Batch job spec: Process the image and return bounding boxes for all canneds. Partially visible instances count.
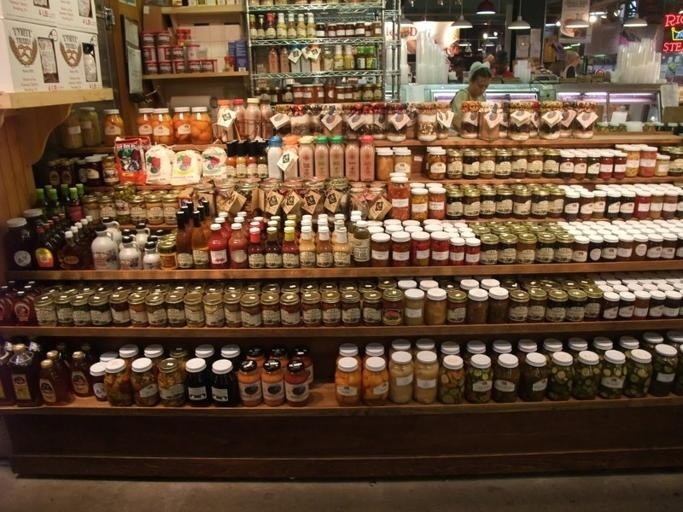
[255,81,597,142]
[5,143,683,407]
[141,29,247,74]
[55,106,213,147]
[315,22,382,39]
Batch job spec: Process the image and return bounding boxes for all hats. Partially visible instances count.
[468,60,491,81]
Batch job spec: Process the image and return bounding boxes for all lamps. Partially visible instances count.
[449,0,647,49]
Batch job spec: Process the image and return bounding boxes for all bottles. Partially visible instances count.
[0,336,93,408]
[48,155,79,187]
[33,181,85,220]
[268,44,376,73]
[174,200,370,270]
[247,12,315,39]
[325,79,335,103]
[31,212,162,272]
[0,280,41,327]
[254,84,292,103]
[226,134,376,184]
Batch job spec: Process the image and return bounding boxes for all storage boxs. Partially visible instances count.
[661,105,682,123]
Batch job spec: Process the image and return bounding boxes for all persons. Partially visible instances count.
[559,49,581,78]
[490,50,514,77]
[450,60,494,110]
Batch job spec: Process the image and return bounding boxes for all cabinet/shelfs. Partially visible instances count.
[140,4,248,78]
[249,2,384,88]
[0,132,683,418]
[400,84,660,128]
